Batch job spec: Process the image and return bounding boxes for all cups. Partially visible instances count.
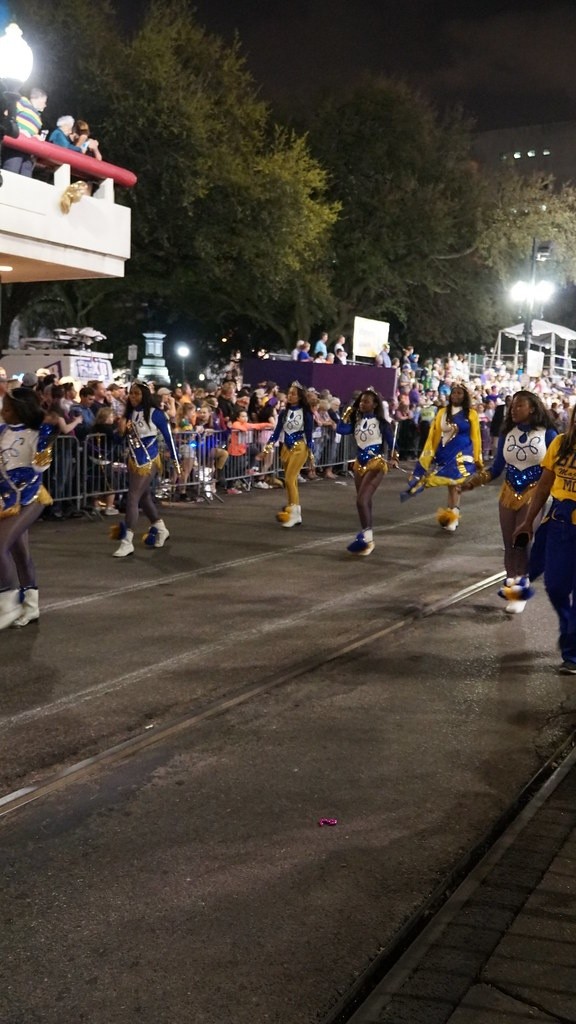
[75,410,82,424]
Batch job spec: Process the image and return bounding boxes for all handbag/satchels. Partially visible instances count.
[112,462,127,473]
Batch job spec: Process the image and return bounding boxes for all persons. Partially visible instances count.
[512,403,576,675]
[456,390,560,614]
[399,384,484,531]
[112,379,181,557]
[335,390,400,555]
[0,388,55,630]
[0,331,576,520]
[3,88,102,195]
[256,380,316,527]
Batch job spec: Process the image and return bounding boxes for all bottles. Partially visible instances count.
[181,417,189,426]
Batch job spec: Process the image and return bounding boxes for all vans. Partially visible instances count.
[1,326,116,389]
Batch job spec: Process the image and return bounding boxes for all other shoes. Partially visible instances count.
[90,506,103,515]
[559,661,576,674]
[155,473,338,503]
[105,507,119,515]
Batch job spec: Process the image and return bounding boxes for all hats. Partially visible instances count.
[30,88,47,99]
[157,388,173,396]
[107,383,123,391]
[22,372,38,386]
[36,368,50,377]
[56,115,75,126]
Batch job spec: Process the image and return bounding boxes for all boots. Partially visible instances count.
[109,522,134,558]
[143,518,169,548]
[347,529,377,556]
[0,587,25,630]
[438,507,460,532]
[497,576,534,614]
[10,585,39,629]
[275,504,302,529]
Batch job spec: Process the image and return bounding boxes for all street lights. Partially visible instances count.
[511,236,557,374]
[175,345,190,383]
